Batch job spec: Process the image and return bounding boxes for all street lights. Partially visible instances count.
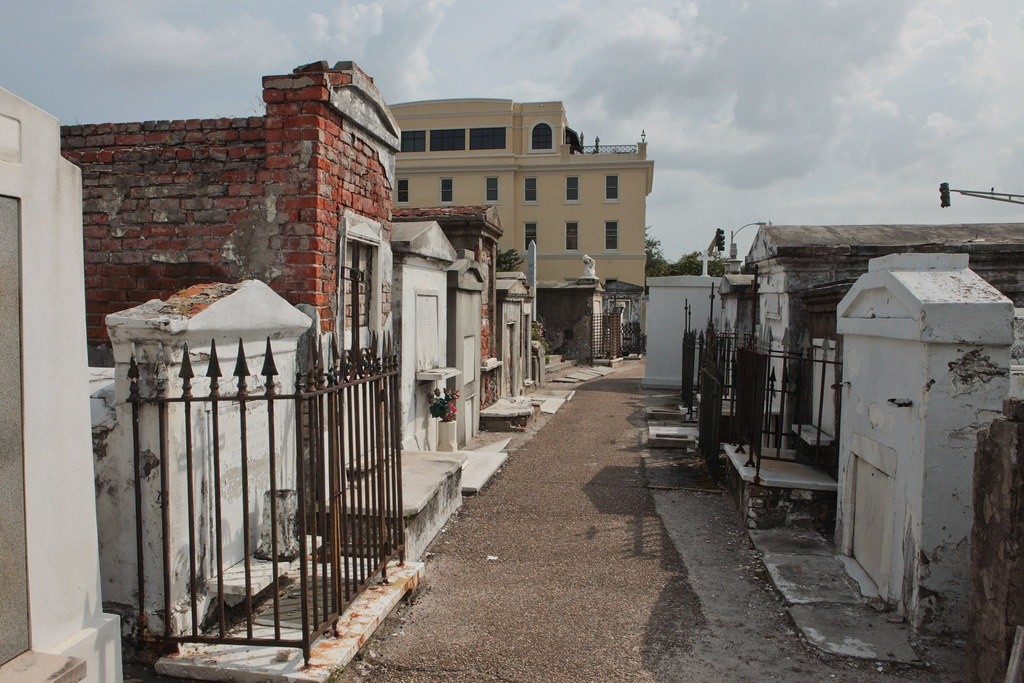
[731,220,772,258]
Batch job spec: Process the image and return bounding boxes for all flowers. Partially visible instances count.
[427,387,461,421]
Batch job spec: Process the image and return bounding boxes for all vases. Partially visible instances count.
[437,420,457,452]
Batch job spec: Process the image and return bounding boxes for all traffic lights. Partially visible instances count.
[715,228,725,252]
[939,182,951,208]
